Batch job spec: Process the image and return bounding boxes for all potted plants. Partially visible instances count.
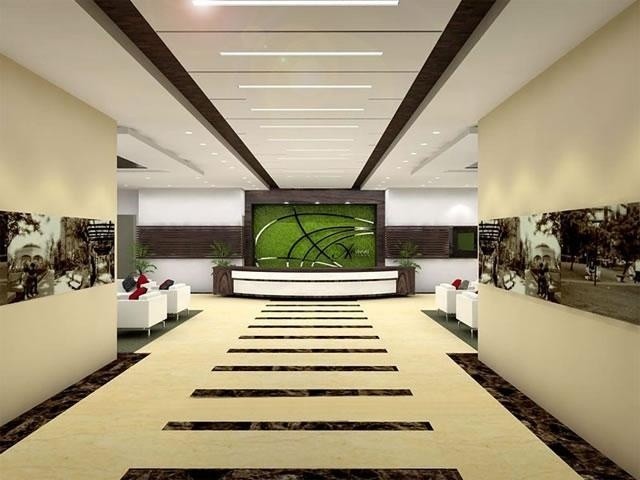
[210,238,235,297]
[391,236,423,296]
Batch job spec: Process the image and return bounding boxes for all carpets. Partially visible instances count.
[420,309,479,353]
[117,309,204,353]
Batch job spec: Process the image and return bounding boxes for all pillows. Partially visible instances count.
[452,278,471,289]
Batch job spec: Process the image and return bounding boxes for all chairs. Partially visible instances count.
[117,277,191,337]
[435,282,478,338]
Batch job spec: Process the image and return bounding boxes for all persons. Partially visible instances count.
[489,241,499,287]
[22,263,39,299]
[589,261,596,280]
[633,255,640,284]
[536,263,550,300]
